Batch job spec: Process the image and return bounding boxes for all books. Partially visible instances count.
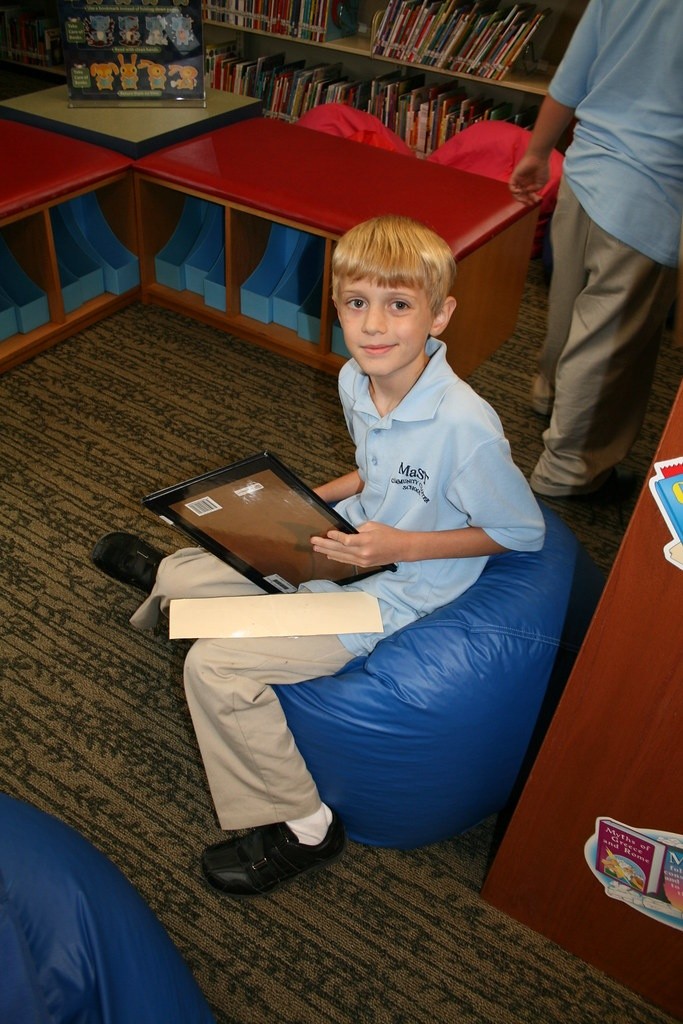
[200,0,553,160]
[0,7,64,67]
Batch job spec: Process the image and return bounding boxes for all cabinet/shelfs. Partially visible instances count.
[1,82,542,388]
[0,1,552,98]
[480,370,683,1024]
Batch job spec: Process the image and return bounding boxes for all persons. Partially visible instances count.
[89,218,545,895]
[508,0,683,496]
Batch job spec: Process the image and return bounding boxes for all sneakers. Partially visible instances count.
[200,801,346,897]
[91,530,173,594]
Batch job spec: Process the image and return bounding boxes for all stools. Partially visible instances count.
[0,791,221,1024]
[265,499,605,849]
[422,118,566,259]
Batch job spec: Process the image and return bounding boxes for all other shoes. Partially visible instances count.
[532,465,636,507]
